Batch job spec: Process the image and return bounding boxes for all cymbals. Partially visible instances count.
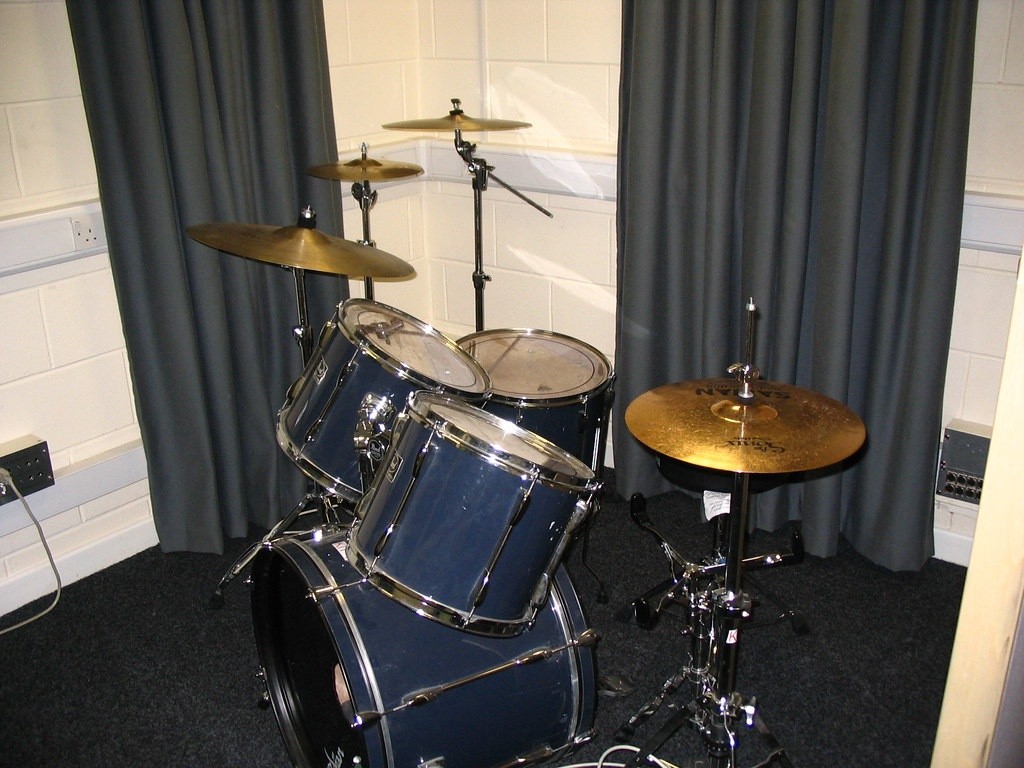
[625,376,867,474]
[381,112,534,133]
[306,158,424,184]
[186,221,414,278]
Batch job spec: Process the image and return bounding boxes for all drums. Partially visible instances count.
[345,389,602,639]
[247,516,600,768]
[449,327,617,480]
[273,299,494,506]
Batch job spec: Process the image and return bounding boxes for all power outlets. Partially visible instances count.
[0,433,55,506]
[70,211,108,251]
